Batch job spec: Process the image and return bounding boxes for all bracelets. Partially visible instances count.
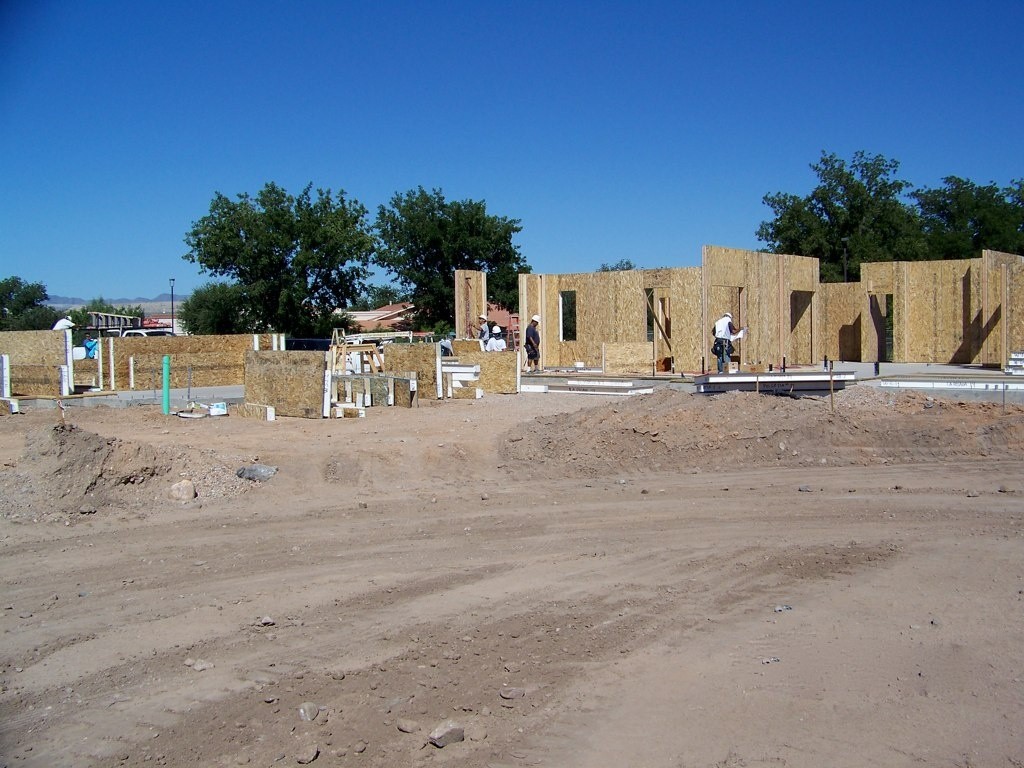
[473,325,475,327]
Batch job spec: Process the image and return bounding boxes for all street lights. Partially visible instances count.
[169,279,175,333]
[841,237,850,282]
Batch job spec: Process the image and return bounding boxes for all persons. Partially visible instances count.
[82,332,98,358]
[449,331,456,340]
[470,314,489,345]
[711,313,744,375]
[51,315,80,330]
[485,325,507,351]
[524,314,541,373]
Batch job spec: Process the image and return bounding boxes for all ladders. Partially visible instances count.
[507,316,519,349]
[87,311,143,330]
[331,328,351,366]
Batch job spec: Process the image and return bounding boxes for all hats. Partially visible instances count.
[724,312,732,318]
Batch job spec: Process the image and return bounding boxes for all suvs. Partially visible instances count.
[122,329,177,337]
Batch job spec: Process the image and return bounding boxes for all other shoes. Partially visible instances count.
[534,370,544,372]
[719,371,723,374]
[525,370,534,374]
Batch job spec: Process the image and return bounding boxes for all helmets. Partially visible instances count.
[479,315,487,321]
[65,316,72,321]
[492,325,501,333]
[532,315,540,323]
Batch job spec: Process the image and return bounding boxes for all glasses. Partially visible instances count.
[85,336,91,337]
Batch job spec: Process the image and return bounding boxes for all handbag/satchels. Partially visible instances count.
[725,345,735,355]
[526,344,531,351]
[712,342,724,357]
